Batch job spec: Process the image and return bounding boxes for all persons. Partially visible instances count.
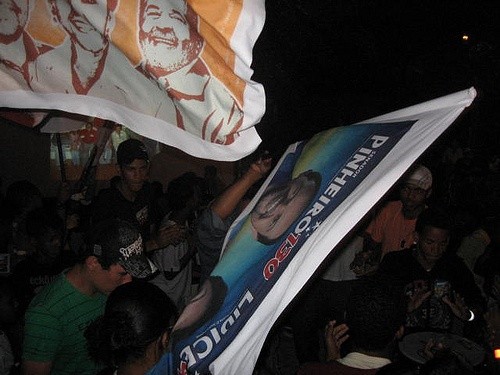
[22,124,271,375]
[292,167,500,375]
[228,143,322,245]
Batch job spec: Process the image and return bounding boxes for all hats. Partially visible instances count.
[407,166,432,191]
[81,217,160,279]
[117,139,149,166]
